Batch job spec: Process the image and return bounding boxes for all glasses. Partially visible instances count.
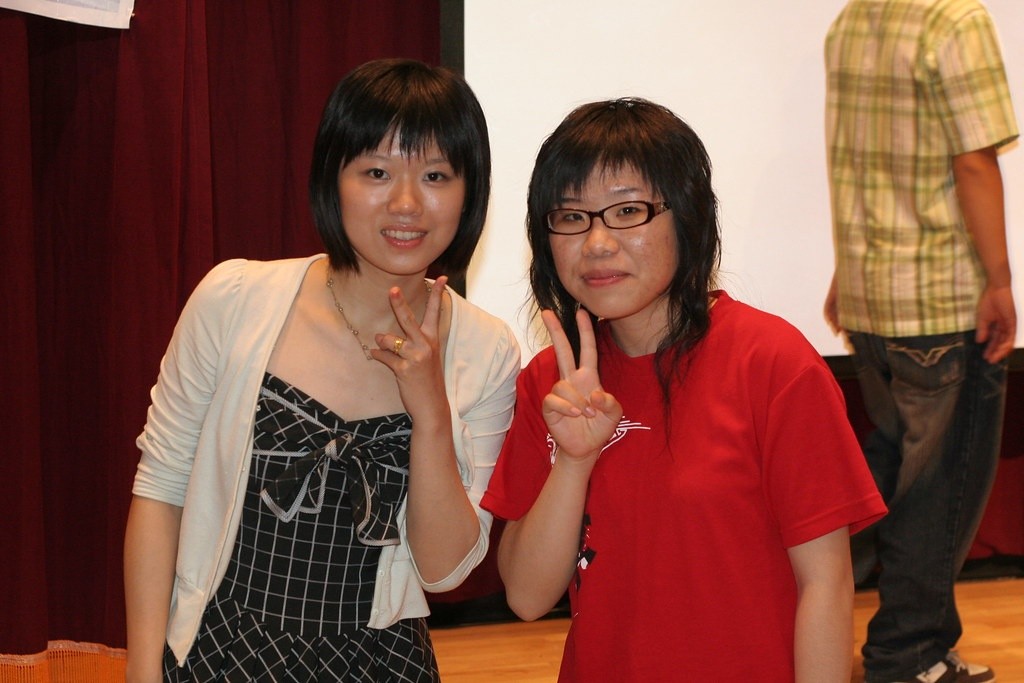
[542,200,673,235]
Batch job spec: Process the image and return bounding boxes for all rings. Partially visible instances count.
[392,337,403,354]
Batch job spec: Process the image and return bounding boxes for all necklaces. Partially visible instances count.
[324,263,431,361]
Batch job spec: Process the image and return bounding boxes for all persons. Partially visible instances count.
[478,97,888,683]
[124,59,522,683]
[823,1,1020,683]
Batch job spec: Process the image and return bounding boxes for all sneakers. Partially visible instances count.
[891,650,997,683]
[854,571,881,591]
